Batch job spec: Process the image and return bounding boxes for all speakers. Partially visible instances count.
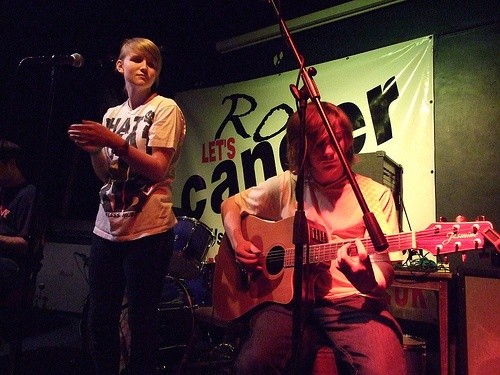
[26,236,97,315]
[448,266,500,375]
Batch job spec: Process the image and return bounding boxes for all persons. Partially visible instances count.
[0,138,45,338]
[66,37,186,375]
[221,102,408,375]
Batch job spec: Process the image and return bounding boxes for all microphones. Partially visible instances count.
[28,53,84,68]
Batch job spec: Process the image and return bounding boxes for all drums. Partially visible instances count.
[167,215,213,281]
[79,268,195,374]
[188,264,216,307]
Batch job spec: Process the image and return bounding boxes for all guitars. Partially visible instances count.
[214,211,500,322]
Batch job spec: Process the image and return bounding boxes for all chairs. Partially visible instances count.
[193,307,248,375]
